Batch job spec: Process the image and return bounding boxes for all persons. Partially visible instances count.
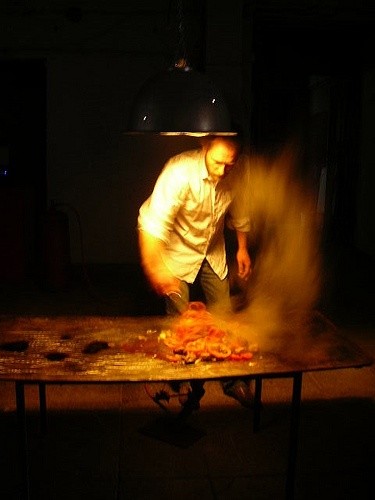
[135,136,253,315]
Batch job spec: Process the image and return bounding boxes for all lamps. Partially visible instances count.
[121,4,237,137]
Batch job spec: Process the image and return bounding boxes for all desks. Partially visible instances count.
[0,309,375,474]
